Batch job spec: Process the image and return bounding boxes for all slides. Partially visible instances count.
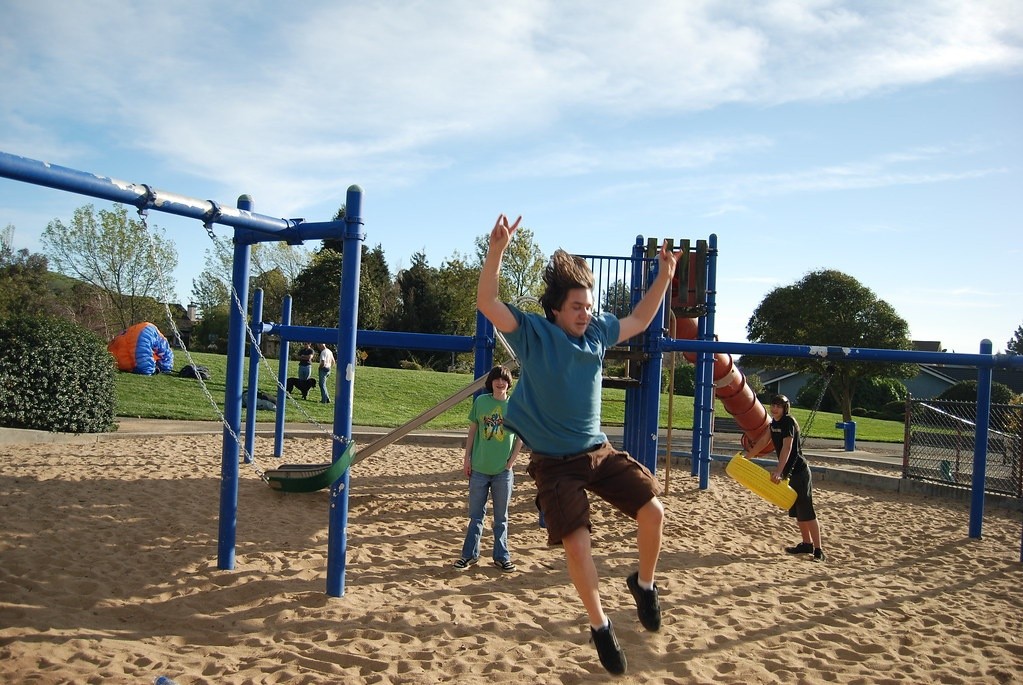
[666,316,776,457]
[263,357,520,479]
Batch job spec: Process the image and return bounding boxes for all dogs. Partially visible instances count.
[287,378,316,400]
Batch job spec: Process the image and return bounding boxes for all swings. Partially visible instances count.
[140,217,357,494]
[724,360,838,512]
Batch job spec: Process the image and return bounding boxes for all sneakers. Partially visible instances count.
[627,572,661,631]
[494,560,516,573]
[785,542,813,554]
[589,617,627,676]
[453,557,479,570]
[813,548,822,562]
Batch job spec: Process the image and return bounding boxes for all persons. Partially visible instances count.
[452,366,524,573]
[317,344,335,403]
[298,343,314,381]
[477,213,684,676]
[745,395,825,563]
